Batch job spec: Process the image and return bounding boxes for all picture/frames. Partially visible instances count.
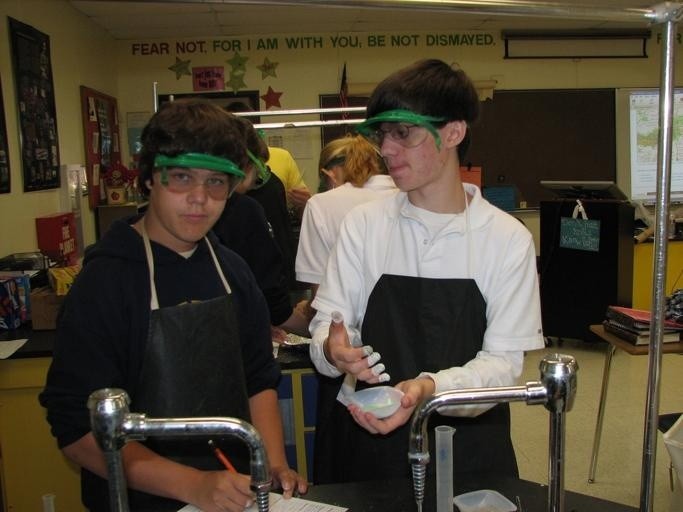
[0,16,60,194]
[158,90,260,123]
[318,93,372,149]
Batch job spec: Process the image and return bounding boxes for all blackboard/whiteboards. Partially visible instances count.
[319,88,616,213]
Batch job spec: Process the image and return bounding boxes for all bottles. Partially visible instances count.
[434,424,457,512]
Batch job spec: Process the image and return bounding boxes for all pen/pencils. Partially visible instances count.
[208,440,238,474]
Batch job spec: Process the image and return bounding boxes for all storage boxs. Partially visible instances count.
[31,287,62,331]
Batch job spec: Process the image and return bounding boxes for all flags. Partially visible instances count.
[338,62,351,119]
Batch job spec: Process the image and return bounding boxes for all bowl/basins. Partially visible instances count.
[347,385,405,420]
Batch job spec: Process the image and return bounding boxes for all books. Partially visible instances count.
[601,304,682,348]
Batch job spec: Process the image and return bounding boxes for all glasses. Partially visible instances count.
[157,157,241,199]
[253,165,271,190]
[366,123,418,143]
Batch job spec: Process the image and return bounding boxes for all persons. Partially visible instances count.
[294,131,404,317]
[223,100,312,225]
[39,95,310,510]
[243,132,305,290]
[305,57,546,479]
[210,118,310,347]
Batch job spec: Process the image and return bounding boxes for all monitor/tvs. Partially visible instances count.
[540,180,628,201]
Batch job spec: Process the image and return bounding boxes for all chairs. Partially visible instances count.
[590,323,683,481]
